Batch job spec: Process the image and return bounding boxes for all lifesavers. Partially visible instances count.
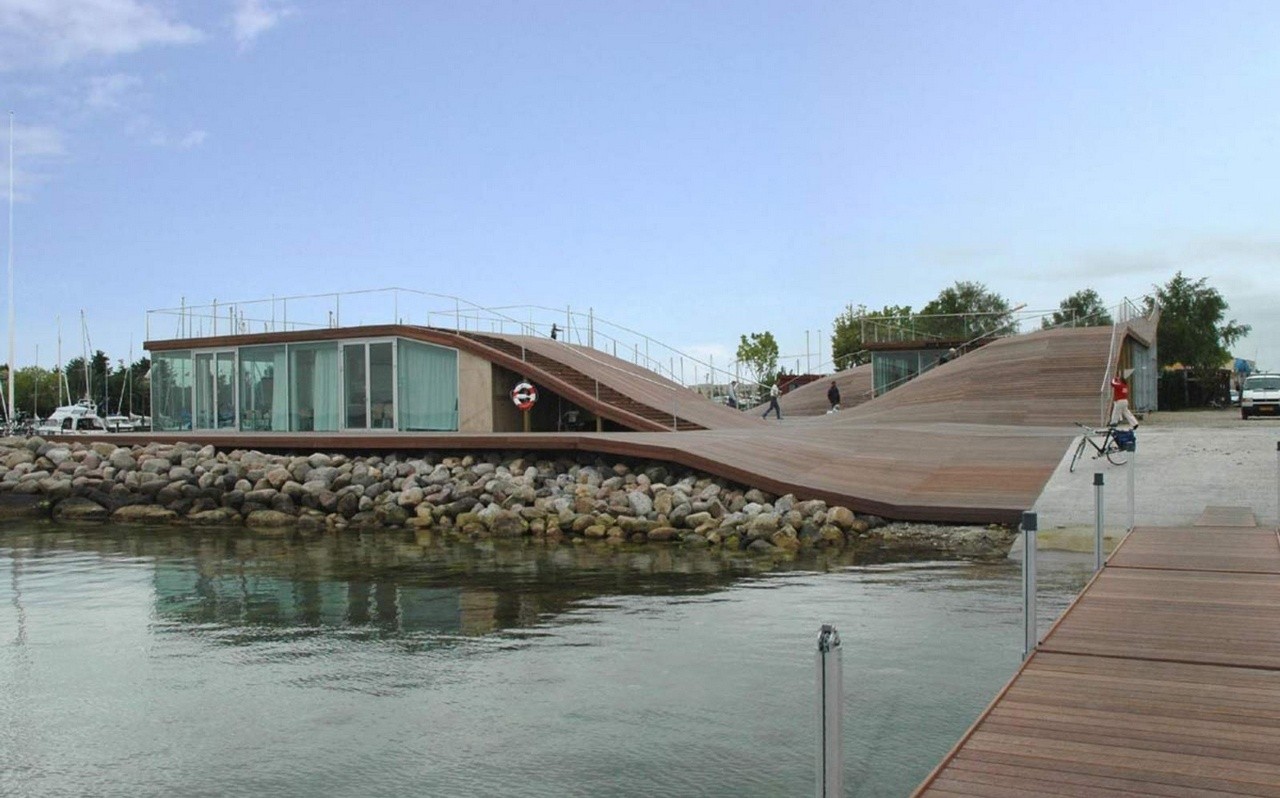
[511,382,538,412]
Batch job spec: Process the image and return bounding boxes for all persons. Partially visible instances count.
[761,378,783,420]
[550,323,562,341]
[728,381,737,408]
[1109,376,1139,431]
[827,382,840,410]
[933,352,949,366]
[948,348,960,360]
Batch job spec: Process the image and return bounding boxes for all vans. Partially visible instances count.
[1235,373,1280,420]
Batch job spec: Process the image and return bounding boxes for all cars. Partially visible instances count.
[1228,389,1241,406]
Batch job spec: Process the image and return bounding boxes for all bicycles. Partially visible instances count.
[1069,422,1137,473]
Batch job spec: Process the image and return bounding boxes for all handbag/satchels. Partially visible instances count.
[1112,430,1136,451]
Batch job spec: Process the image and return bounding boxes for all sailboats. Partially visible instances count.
[0,309,271,436]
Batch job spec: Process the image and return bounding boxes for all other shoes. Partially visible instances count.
[1108,424,1116,428]
[1133,424,1138,430]
